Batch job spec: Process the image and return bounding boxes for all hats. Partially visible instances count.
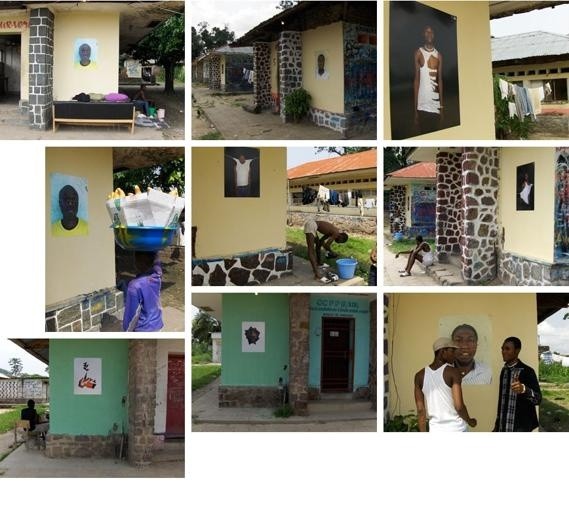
[432,337,459,352]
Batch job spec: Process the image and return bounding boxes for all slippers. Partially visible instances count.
[317,262,330,269]
[399,269,411,277]
[315,276,332,284]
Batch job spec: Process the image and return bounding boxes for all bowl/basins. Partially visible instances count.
[109,225,178,253]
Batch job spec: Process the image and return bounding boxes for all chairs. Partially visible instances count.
[13,414,48,450]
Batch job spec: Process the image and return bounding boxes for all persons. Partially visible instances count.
[414,338,477,432]
[52,185,89,235]
[21,400,39,435]
[314,54,329,80]
[449,323,493,385]
[395,235,434,277]
[412,23,444,132]
[123,251,163,332]
[74,43,96,73]
[304,219,348,284]
[234,154,251,197]
[490,337,542,431]
[517,172,534,210]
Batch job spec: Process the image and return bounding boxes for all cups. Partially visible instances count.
[148,108,165,119]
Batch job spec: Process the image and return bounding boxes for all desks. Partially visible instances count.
[51,100,136,137]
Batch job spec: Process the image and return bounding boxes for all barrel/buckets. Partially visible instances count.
[335,258,358,279]
[395,232,403,241]
